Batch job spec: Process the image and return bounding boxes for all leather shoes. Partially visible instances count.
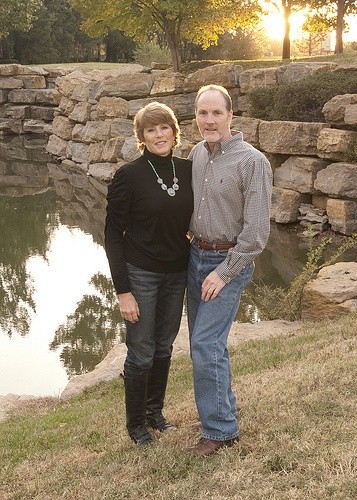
[183,436,239,458]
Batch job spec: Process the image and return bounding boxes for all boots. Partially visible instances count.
[146,355,179,431]
[124,364,153,447]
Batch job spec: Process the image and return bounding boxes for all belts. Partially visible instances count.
[195,239,237,250]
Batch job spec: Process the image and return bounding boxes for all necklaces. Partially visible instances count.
[148,156,179,198]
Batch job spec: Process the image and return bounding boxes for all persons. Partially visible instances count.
[189,85,271,462]
[105,102,193,445]
